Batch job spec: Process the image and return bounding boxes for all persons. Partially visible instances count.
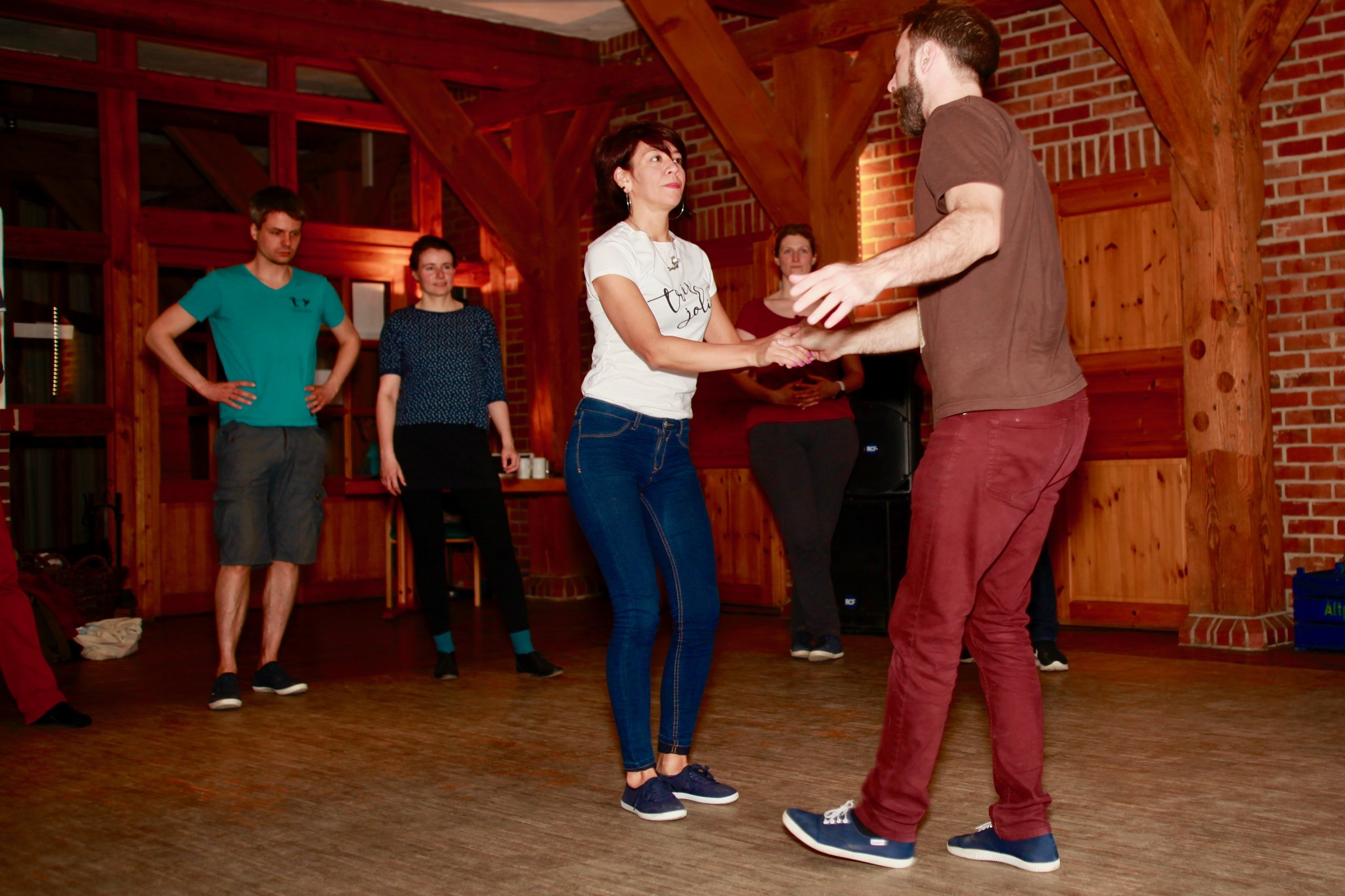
[563,123,812,822]
[145,184,360,710]
[735,224,863,659]
[0,498,92,727]
[777,0,1091,874]
[376,235,563,677]
[960,537,1070,671]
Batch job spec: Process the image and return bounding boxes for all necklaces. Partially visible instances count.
[627,219,680,271]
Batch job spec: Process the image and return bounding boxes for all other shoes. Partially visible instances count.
[31,702,92,728]
[514,652,564,677]
[434,651,459,680]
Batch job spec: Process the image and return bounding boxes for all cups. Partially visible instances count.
[532,457,546,480]
[517,455,531,479]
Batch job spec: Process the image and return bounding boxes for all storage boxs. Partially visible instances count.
[1293,560,1345,652]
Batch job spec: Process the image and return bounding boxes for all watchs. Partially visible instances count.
[832,381,845,399]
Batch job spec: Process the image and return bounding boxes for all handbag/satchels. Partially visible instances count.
[76,616,142,660]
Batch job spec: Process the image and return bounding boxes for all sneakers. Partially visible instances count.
[960,647,976,664]
[208,672,243,710]
[789,643,809,658]
[782,800,915,868]
[1033,641,1068,671]
[251,660,307,695]
[655,762,740,805]
[947,822,1060,872]
[808,636,844,662]
[620,777,687,821]
